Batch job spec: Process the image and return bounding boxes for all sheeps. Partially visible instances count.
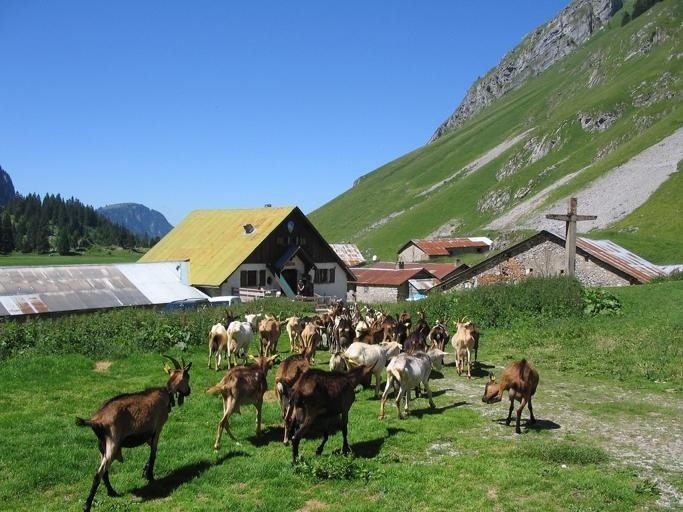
[482,359,539,434]
[75,355,192,512]
[208,301,480,466]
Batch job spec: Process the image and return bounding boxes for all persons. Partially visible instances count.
[298,279,304,295]
